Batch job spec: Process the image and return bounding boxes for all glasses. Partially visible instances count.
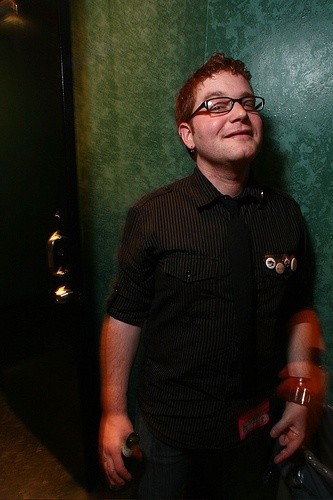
[187,95,266,120]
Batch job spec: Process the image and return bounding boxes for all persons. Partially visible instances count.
[96,52,326,500]
